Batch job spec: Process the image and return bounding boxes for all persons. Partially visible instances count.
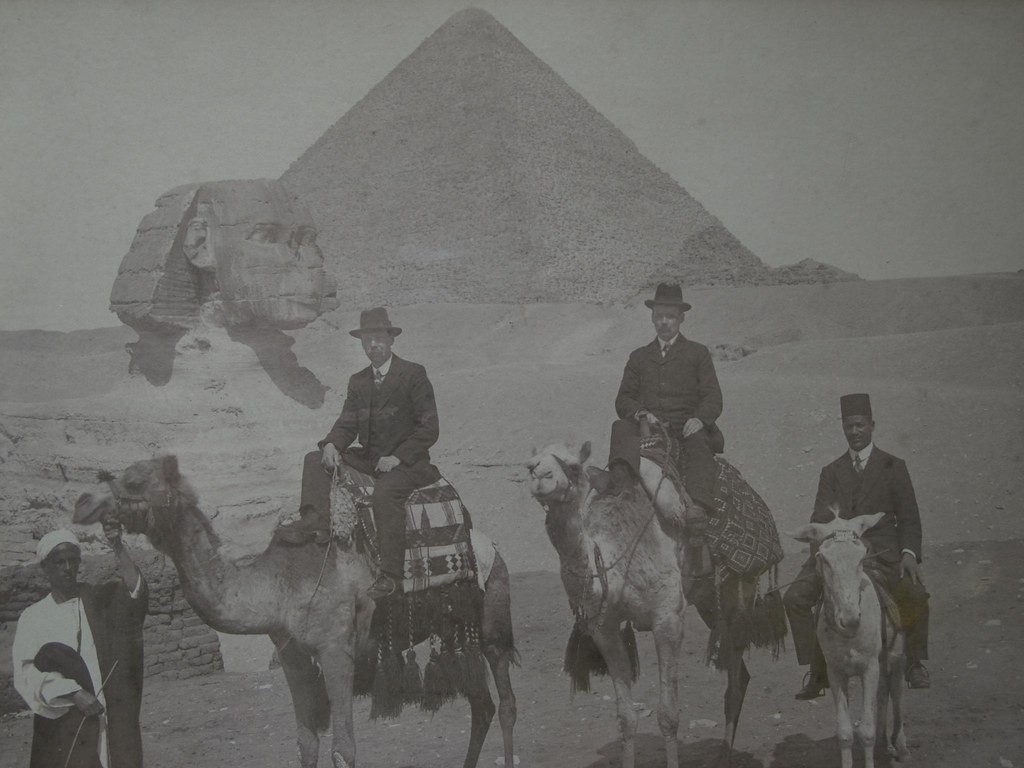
[12,518,149,768]
[279,307,439,599]
[109,180,326,332]
[784,393,931,699]
[589,282,723,547]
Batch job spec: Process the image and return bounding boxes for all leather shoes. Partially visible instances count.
[375,570,405,593]
[274,509,331,545]
[795,663,830,699]
[904,661,929,690]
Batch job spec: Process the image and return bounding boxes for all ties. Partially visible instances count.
[852,455,865,480]
[375,370,382,387]
[664,343,672,356]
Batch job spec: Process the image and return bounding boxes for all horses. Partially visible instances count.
[790,506,912,768]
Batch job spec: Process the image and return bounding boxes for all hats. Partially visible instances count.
[645,283,691,312]
[840,394,872,417]
[349,307,402,339]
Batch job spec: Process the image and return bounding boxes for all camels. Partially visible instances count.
[524,441,790,768]
[71,453,522,768]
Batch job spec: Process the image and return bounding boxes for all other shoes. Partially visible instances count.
[586,462,634,499]
[688,504,705,548]
